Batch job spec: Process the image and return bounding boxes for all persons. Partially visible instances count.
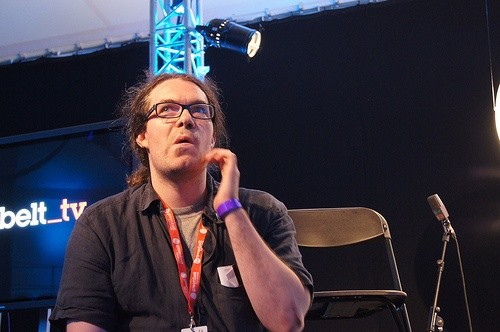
[49,73,315,332]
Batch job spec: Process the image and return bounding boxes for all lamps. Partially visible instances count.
[195,18,262,58]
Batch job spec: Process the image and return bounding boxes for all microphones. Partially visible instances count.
[428,192,455,240]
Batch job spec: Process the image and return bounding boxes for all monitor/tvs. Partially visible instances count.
[0,116,139,311]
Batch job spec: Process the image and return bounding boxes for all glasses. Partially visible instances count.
[144,101,216,119]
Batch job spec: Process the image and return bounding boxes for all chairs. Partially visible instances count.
[288,207,412,332]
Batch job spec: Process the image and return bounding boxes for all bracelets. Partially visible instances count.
[216,199,242,221]
